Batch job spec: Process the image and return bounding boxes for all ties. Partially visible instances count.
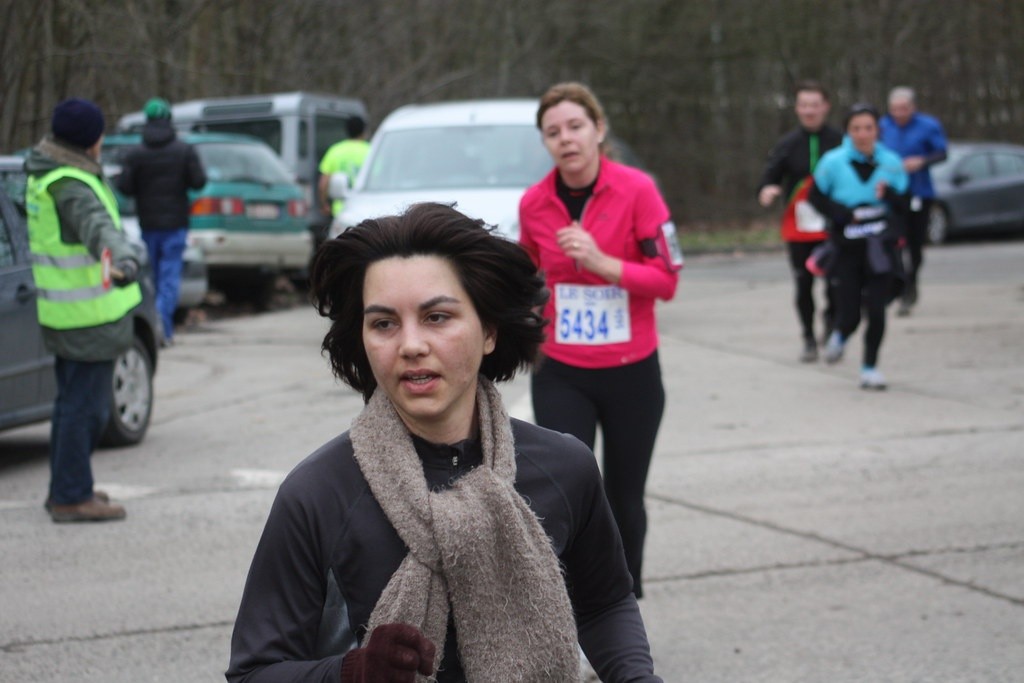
[808,136,819,174]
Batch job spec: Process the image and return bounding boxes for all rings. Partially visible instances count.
[573,242,580,248]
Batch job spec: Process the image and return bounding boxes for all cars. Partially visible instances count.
[93,134,316,298]
[1,156,168,447]
[924,139,1024,245]
[325,100,553,256]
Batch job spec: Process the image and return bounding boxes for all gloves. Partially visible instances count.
[341,623,436,683]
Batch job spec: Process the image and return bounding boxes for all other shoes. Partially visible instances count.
[45,491,113,513]
[161,339,174,348]
[801,345,818,362]
[51,499,126,523]
[896,304,910,318]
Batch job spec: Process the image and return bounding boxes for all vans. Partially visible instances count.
[113,90,368,222]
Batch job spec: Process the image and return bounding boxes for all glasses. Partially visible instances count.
[848,102,874,113]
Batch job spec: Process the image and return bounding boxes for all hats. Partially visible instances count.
[51,98,105,150]
[143,97,170,118]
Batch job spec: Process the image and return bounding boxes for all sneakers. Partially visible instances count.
[823,341,843,365]
[858,365,889,390]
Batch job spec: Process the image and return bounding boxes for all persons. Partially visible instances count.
[114,97,207,345]
[517,82,684,601]
[756,81,844,361]
[806,103,909,391]
[877,86,947,319]
[317,115,371,223]
[23,99,143,523]
[223,204,664,683]
